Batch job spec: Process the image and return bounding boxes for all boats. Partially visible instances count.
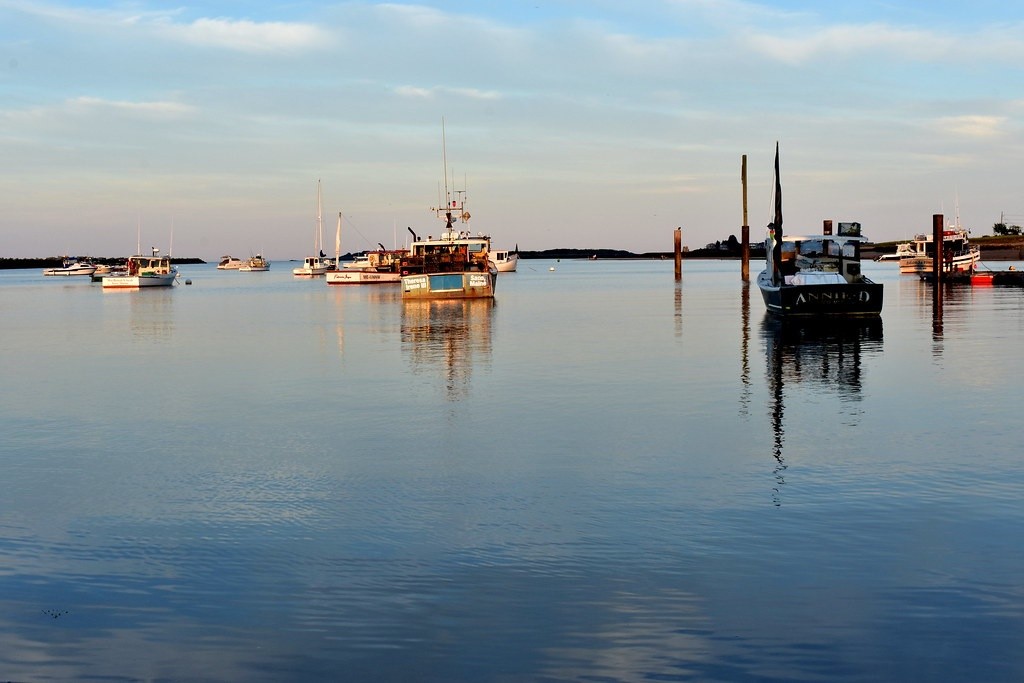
[877,242,917,261]
[218,248,272,272]
[898,207,981,278]
[490,249,519,273]
[755,139,885,318]
[41,247,180,289]
[400,228,499,301]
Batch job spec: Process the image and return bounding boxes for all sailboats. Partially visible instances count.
[326,210,409,285]
[290,174,336,275]
